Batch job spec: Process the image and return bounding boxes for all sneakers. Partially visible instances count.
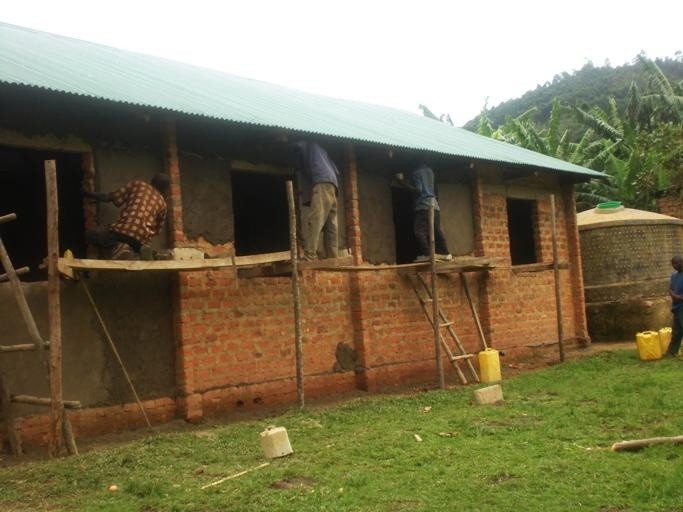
[412,255,431,262]
[662,349,677,358]
[109,243,129,260]
[303,251,318,262]
[139,242,155,261]
[435,252,453,261]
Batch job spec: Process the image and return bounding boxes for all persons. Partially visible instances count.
[271,133,340,262]
[80,166,171,260]
[662,255,683,355]
[392,160,453,262]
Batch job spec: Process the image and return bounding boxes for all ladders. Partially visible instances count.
[0,212,81,457]
[407,271,481,385]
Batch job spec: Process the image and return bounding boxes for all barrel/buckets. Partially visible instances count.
[479,348,501,384]
[659,326,672,353]
[635,331,662,361]
